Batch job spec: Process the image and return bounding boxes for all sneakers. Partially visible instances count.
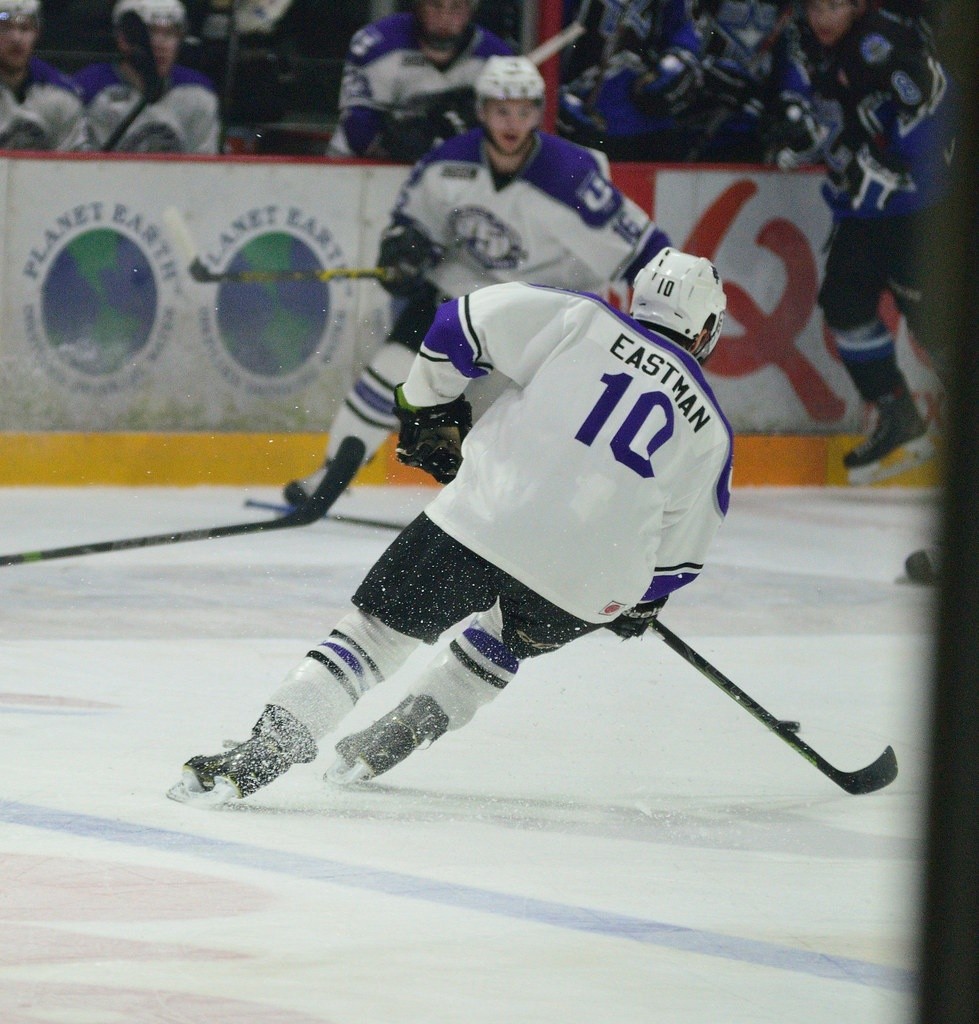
[323,693,450,786]
[167,703,318,810]
[843,380,935,486]
[285,459,335,509]
[896,546,941,585]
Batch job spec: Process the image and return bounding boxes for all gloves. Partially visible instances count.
[821,135,916,218]
[766,101,820,170]
[604,596,668,640]
[393,381,473,485]
[376,212,440,296]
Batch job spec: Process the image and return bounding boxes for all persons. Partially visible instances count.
[172,244,733,812]
[742,0,965,475]
[283,52,673,513]
[0,0,775,159]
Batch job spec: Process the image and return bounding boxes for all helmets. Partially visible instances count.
[0,0,42,33]
[111,0,187,46]
[632,247,727,362]
[476,54,545,119]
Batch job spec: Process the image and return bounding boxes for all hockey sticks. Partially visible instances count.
[641,614,905,800]
[164,203,393,287]
[0,432,367,571]
